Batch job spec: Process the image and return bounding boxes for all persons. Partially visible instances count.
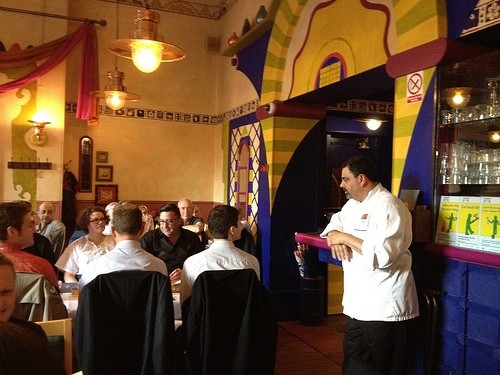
[68,208,92,280]
[180,205,260,305]
[102,202,120,236]
[78,203,167,291]
[319,156,419,375]
[55,206,116,284]
[139,206,154,237]
[178,198,205,248]
[13,200,58,273]
[35,201,66,256]
[0,202,59,287]
[0,253,48,340]
[140,202,202,281]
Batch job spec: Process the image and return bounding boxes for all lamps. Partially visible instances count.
[88,0,143,111]
[477,122,500,144]
[440,59,488,110]
[25,107,52,145]
[105,0,187,73]
[354,105,389,130]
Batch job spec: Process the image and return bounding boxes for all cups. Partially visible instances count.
[440,141,500,185]
[170,280,180,296]
[71,286,79,300]
[441,74,500,124]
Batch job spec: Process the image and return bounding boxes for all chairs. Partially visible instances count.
[181,268,280,375]
[8,271,79,374]
[72,270,178,375]
[33,317,73,375]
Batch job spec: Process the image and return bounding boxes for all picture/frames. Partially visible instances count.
[95,165,113,183]
[94,184,119,207]
[95,151,108,164]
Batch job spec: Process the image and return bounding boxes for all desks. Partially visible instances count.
[58,282,183,320]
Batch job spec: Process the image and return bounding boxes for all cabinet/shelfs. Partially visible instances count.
[436,42,500,198]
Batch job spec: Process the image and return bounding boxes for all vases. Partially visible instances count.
[227,31,240,46]
[255,5,267,24]
[241,18,251,36]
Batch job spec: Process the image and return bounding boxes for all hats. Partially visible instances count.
[105,202,118,211]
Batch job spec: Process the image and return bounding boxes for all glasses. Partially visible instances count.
[88,218,106,224]
[158,216,180,224]
